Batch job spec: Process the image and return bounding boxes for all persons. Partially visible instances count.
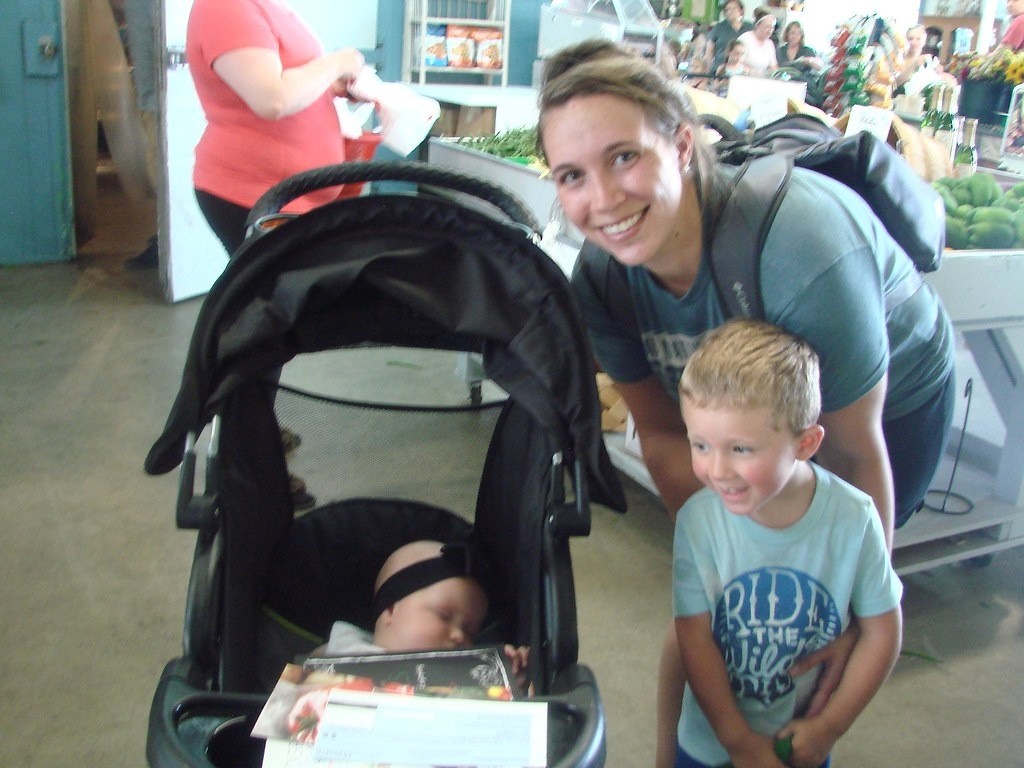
[733,7,780,79]
[107,1,169,272]
[180,1,380,263]
[701,0,757,88]
[775,21,824,80]
[677,40,693,70]
[946,1,1024,156]
[640,311,909,768]
[893,26,940,98]
[685,32,708,68]
[710,39,756,99]
[502,38,960,744]
[307,538,547,685]
[644,39,656,60]
[660,36,685,78]
[706,21,720,35]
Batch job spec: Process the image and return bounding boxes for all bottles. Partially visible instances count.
[920,83,980,176]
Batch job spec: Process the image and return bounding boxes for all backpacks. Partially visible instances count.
[691,103,948,348]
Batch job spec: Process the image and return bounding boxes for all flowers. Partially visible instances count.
[947,44,1024,86]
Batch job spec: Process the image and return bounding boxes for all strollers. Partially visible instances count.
[145,160,627,768]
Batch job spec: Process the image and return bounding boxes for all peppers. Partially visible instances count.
[933,171,1024,249]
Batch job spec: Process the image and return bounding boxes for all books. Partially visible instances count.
[300,671,550,768]
[261,643,522,767]
[248,654,414,746]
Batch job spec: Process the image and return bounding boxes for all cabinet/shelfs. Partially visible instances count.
[402,0,511,90]
